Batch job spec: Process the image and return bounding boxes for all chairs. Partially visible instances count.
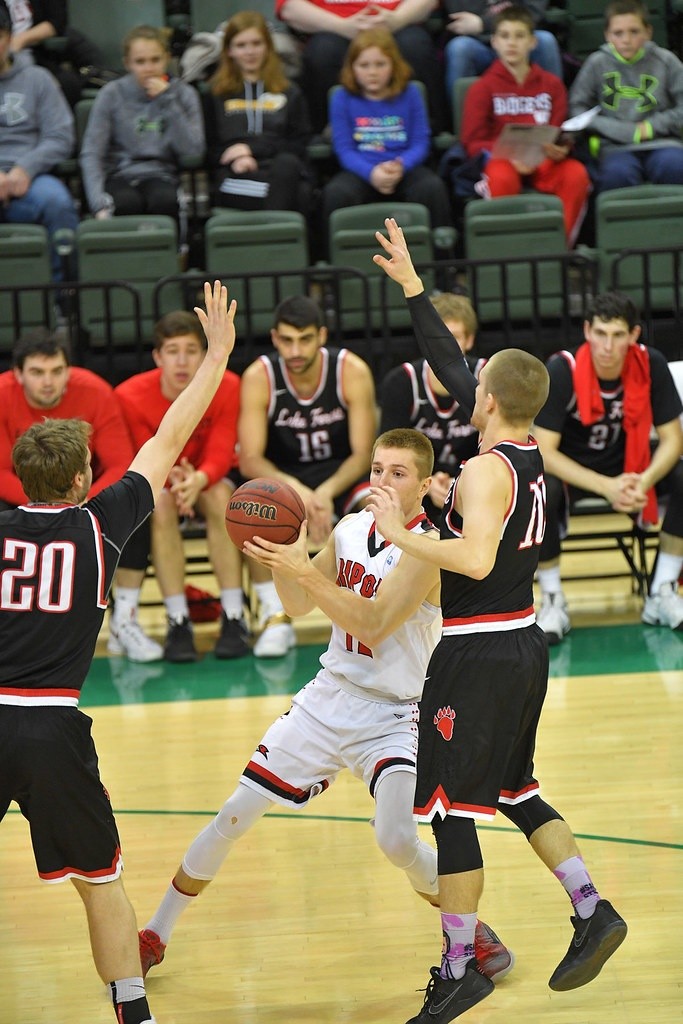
[0,0,683,348]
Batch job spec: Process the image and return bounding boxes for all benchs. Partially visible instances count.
[145,489,668,602]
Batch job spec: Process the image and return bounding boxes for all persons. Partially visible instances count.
[138,428,516,979]
[379,290,514,536]
[530,291,682,643]
[113,309,253,664]
[373,217,627,1023]
[0,278,237,1024]
[0,0,682,323]
[0,337,164,664]
[239,294,376,657]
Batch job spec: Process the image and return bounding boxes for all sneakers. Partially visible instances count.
[536,590,572,643]
[549,900,627,991]
[138,930,166,980]
[106,613,163,662]
[642,581,683,631]
[214,607,253,658]
[253,604,296,657]
[405,957,495,1024]
[163,614,198,660]
[473,919,514,980]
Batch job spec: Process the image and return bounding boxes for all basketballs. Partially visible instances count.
[225,478,309,553]
